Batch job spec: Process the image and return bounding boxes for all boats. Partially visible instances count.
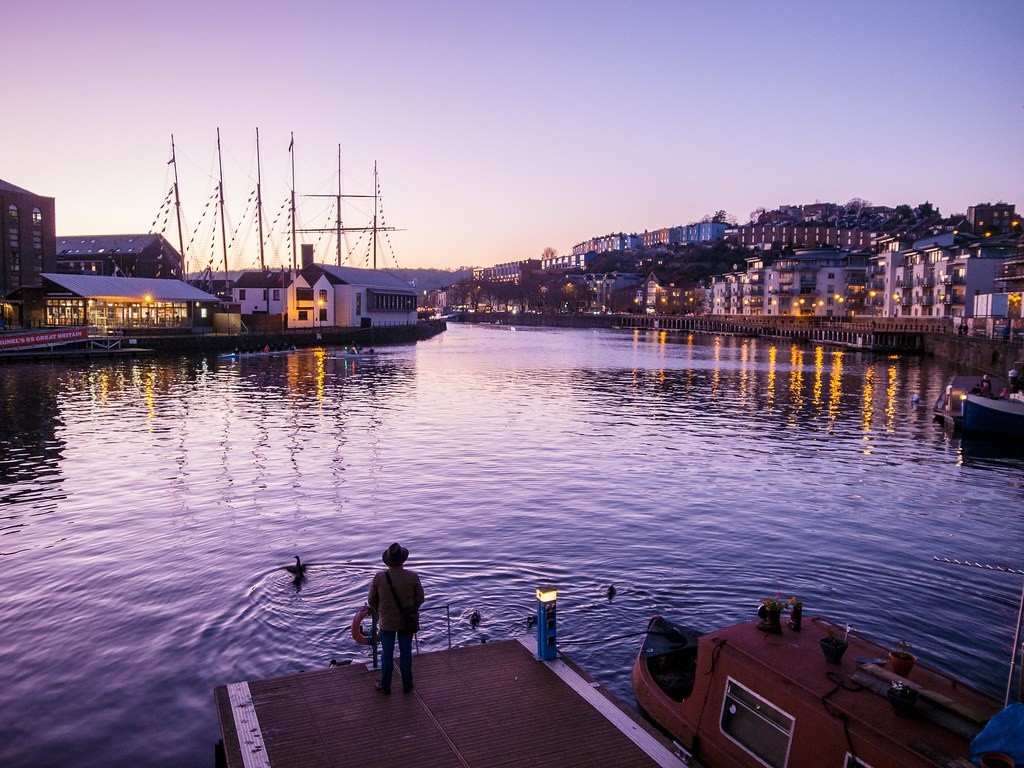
[960,389,1024,431]
[631,590,1024,768]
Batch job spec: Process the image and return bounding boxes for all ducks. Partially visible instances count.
[470,609,481,630]
[286,554,306,591]
[329,659,354,668]
[607,584,616,599]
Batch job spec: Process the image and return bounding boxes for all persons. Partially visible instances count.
[367,542,425,693]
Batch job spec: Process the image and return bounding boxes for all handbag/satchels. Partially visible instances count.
[404,611,420,633]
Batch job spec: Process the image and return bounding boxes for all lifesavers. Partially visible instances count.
[352,607,380,646]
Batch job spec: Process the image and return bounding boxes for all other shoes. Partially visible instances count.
[403,683,414,693]
[375,680,391,695]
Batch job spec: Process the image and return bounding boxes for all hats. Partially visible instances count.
[383,543,409,566]
